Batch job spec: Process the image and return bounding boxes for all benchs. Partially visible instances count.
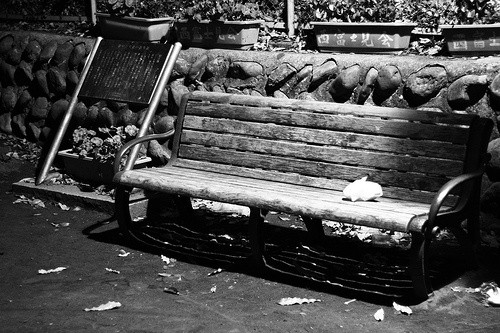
[112,90,494,298]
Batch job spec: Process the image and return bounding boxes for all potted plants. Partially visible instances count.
[58,126,151,183]
[94,0,173,44]
[175,0,264,50]
[437,0,499,57]
[309,0,415,56]
